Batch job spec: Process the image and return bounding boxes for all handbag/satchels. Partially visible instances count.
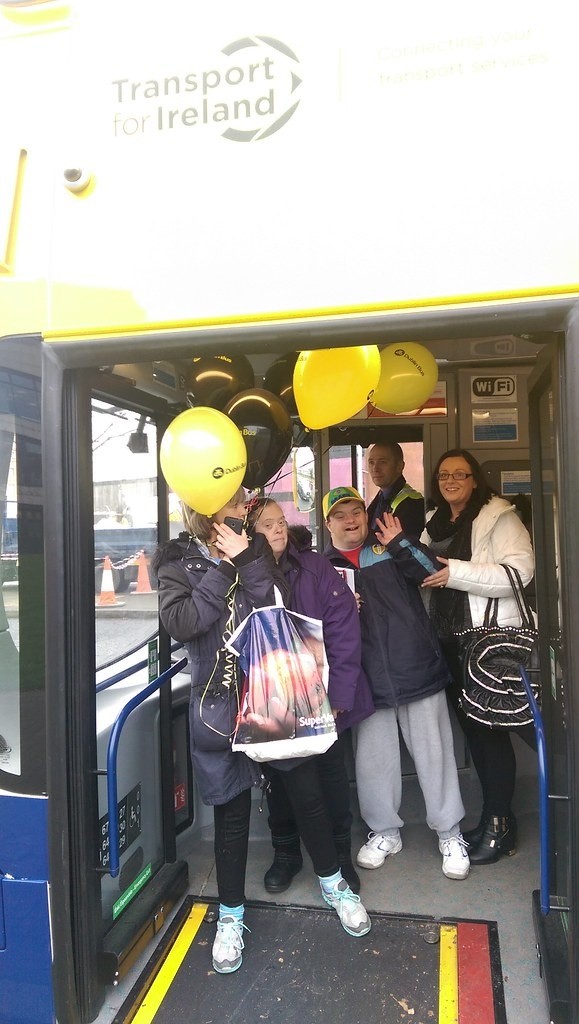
[455,563,542,729]
[224,583,338,761]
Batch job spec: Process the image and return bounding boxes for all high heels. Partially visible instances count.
[466,816,515,864]
[461,810,495,844]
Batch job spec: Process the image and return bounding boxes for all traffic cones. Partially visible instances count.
[132,549,156,594]
[95,555,126,608]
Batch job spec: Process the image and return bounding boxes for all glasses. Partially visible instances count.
[436,472,475,481]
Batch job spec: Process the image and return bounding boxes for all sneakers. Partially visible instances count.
[356,830,403,869]
[211,915,251,974]
[438,834,471,880]
[318,879,371,937]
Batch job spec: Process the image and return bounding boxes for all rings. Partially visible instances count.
[440,585,443,588]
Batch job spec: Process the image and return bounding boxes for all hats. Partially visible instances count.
[323,486,365,522]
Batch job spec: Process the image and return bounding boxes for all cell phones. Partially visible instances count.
[217,516,244,553]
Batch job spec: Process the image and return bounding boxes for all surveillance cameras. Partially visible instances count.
[60,162,91,191]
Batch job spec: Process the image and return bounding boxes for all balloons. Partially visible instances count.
[221,388,293,493]
[369,342,438,414]
[262,353,309,446]
[293,344,381,433]
[160,406,247,518]
[176,354,255,411]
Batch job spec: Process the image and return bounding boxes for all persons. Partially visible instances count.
[365,440,426,539]
[418,450,535,865]
[149,486,371,973]
[247,496,361,896]
[319,485,470,879]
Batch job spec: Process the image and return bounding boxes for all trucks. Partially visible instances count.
[1,499,159,593]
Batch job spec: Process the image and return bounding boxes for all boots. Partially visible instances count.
[263,830,303,892]
[328,831,360,894]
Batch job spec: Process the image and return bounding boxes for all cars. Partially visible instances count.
[297,477,313,501]
